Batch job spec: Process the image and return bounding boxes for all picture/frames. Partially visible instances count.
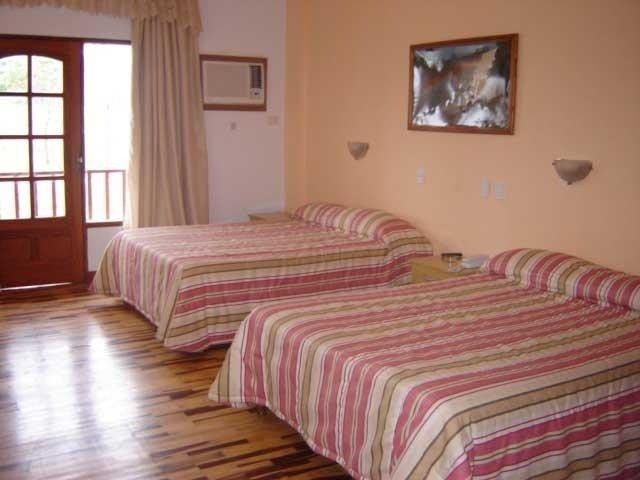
[406,33,519,135]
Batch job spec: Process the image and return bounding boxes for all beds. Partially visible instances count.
[200,250,640,480]
[84,201,434,355]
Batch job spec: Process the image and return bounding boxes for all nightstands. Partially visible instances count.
[406,251,485,289]
[250,210,296,223]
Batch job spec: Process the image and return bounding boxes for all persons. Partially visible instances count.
[410,64,508,129]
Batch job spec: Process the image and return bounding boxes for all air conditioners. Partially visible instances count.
[203,61,266,106]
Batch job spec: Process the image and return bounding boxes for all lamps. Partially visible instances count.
[348,141,369,160]
[551,157,593,186]
